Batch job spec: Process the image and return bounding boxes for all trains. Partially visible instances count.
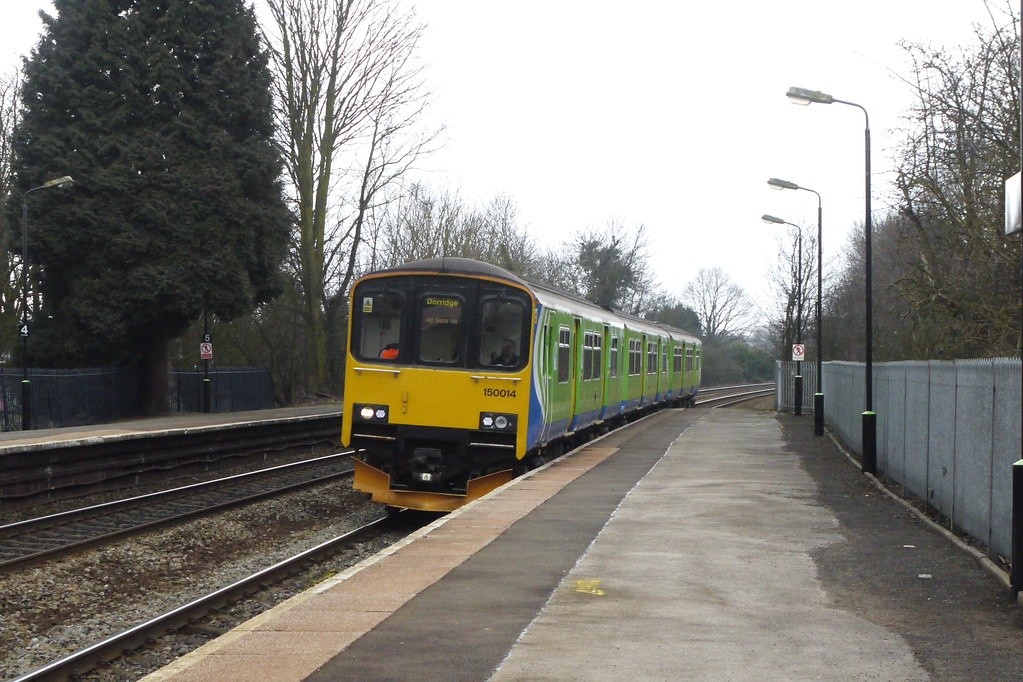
[339,256,703,519]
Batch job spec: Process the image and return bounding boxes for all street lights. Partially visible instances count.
[762,214,804,417]
[787,87,879,473]
[767,176,826,438]
[20,177,77,430]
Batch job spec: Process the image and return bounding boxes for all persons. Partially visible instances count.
[489,339,520,367]
